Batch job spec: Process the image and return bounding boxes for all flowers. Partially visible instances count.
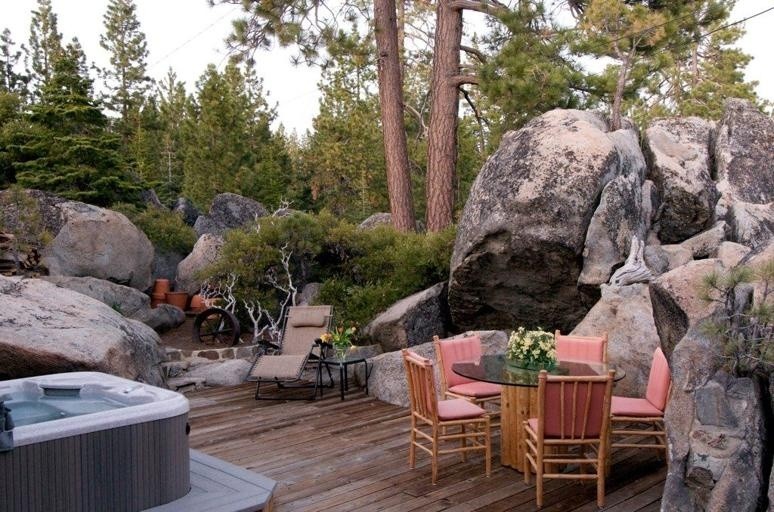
[504,327,558,363]
[320,326,356,349]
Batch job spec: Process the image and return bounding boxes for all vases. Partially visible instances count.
[152,278,221,319]
[335,349,347,361]
[507,357,555,370]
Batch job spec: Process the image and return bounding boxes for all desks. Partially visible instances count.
[452,353,627,475]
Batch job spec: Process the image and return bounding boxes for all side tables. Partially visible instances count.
[319,357,368,402]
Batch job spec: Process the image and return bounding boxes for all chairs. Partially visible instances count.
[245,306,334,401]
[585,346,674,471]
[522,369,615,509]
[402,350,493,486]
[432,335,504,461]
[552,330,608,365]
